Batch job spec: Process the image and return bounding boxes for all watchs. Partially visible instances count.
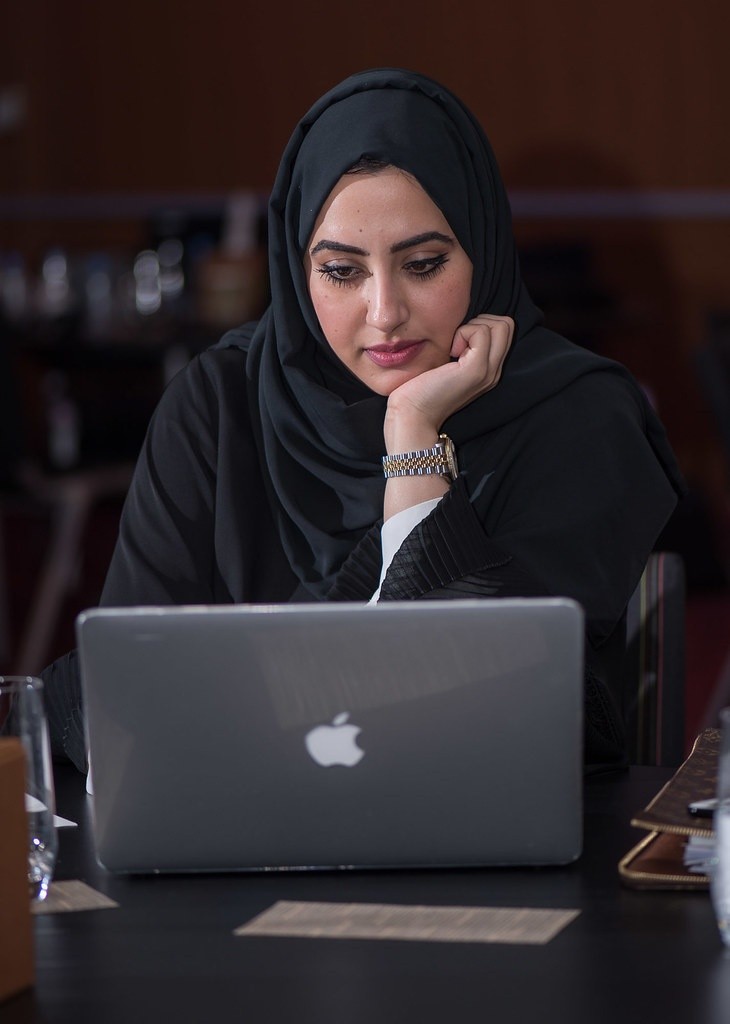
[383,433,463,485]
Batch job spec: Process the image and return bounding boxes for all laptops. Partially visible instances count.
[75,598,585,874]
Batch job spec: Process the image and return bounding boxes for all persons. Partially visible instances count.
[90,68,680,807]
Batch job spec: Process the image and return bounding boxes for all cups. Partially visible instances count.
[0,675,58,904]
